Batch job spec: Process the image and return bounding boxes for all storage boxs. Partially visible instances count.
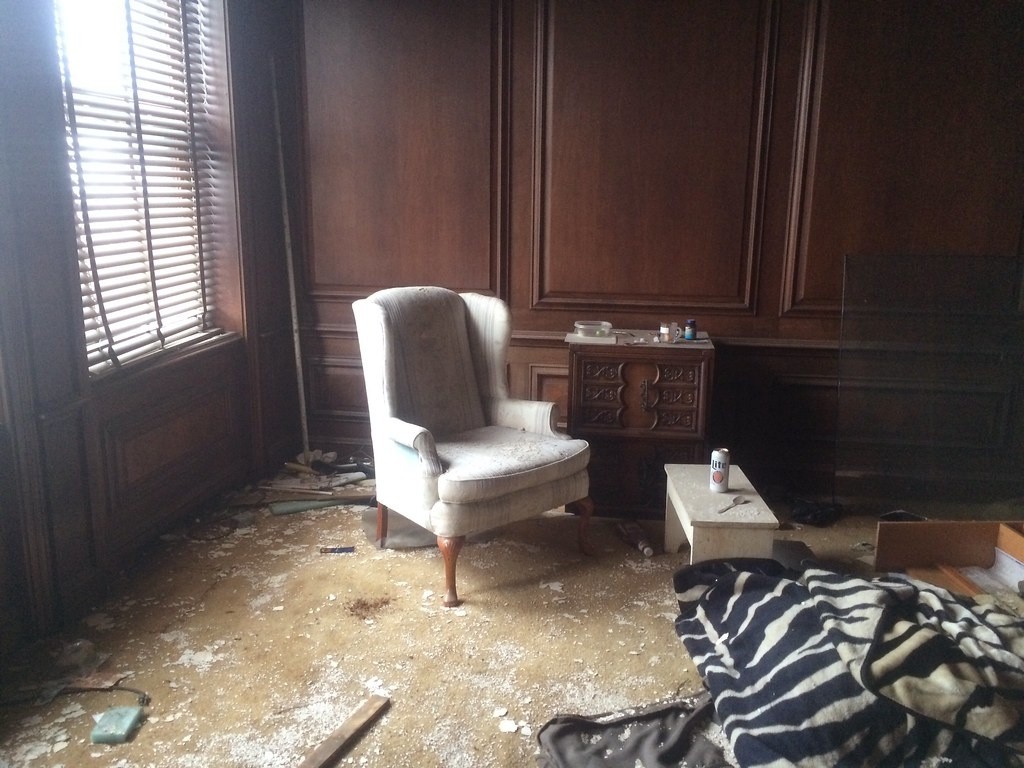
[573,321,612,337]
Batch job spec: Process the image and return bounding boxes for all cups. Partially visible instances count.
[660,324,672,343]
[670,323,681,343]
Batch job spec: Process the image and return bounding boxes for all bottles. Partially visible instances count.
[615,519,652,556]
[684,320,697,339]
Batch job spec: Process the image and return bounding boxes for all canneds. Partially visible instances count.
[710,448,730,493]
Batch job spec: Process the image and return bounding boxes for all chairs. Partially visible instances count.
[352,286,594,608]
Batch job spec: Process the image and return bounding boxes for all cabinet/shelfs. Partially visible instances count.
[566,328,715,517]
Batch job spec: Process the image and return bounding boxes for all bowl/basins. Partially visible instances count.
[574,321,613,337]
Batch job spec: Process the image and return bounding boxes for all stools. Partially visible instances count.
[662,463,780,567]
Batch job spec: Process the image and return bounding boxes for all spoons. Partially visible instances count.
[719,496,744,513]
